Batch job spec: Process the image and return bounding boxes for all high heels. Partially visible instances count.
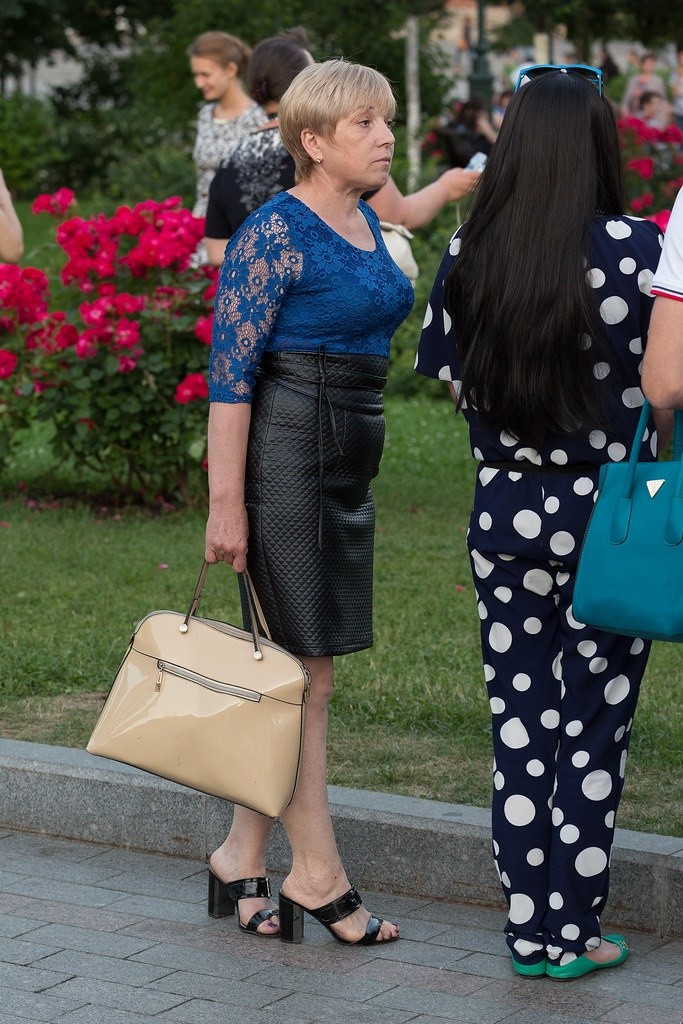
[279,884,400,945]
[207,864,280,938]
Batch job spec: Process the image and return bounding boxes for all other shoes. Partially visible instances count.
[509,950,545,979]
[545,933,629,982]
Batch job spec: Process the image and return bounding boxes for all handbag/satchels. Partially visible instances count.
[85,561,311,818]
[571,396,683,643]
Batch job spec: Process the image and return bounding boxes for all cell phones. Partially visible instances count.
[465,151,489,173]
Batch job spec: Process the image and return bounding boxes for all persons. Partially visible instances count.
[186,32,270,267]
[203,54,414,946]
[0,169,24,263]
[413,74,668,978]
[434,48,683,170]
[639,182,683,408]
[204,33,482,266]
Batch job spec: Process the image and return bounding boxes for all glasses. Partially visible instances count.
[515,63,605,98]
